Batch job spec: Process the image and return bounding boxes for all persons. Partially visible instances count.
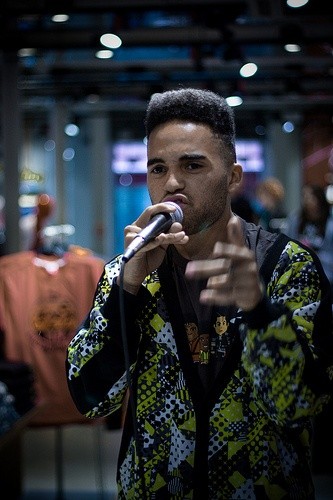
[230,179,333,279]
[64,88,333,500]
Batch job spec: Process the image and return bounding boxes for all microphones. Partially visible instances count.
[122,201,184,262]
